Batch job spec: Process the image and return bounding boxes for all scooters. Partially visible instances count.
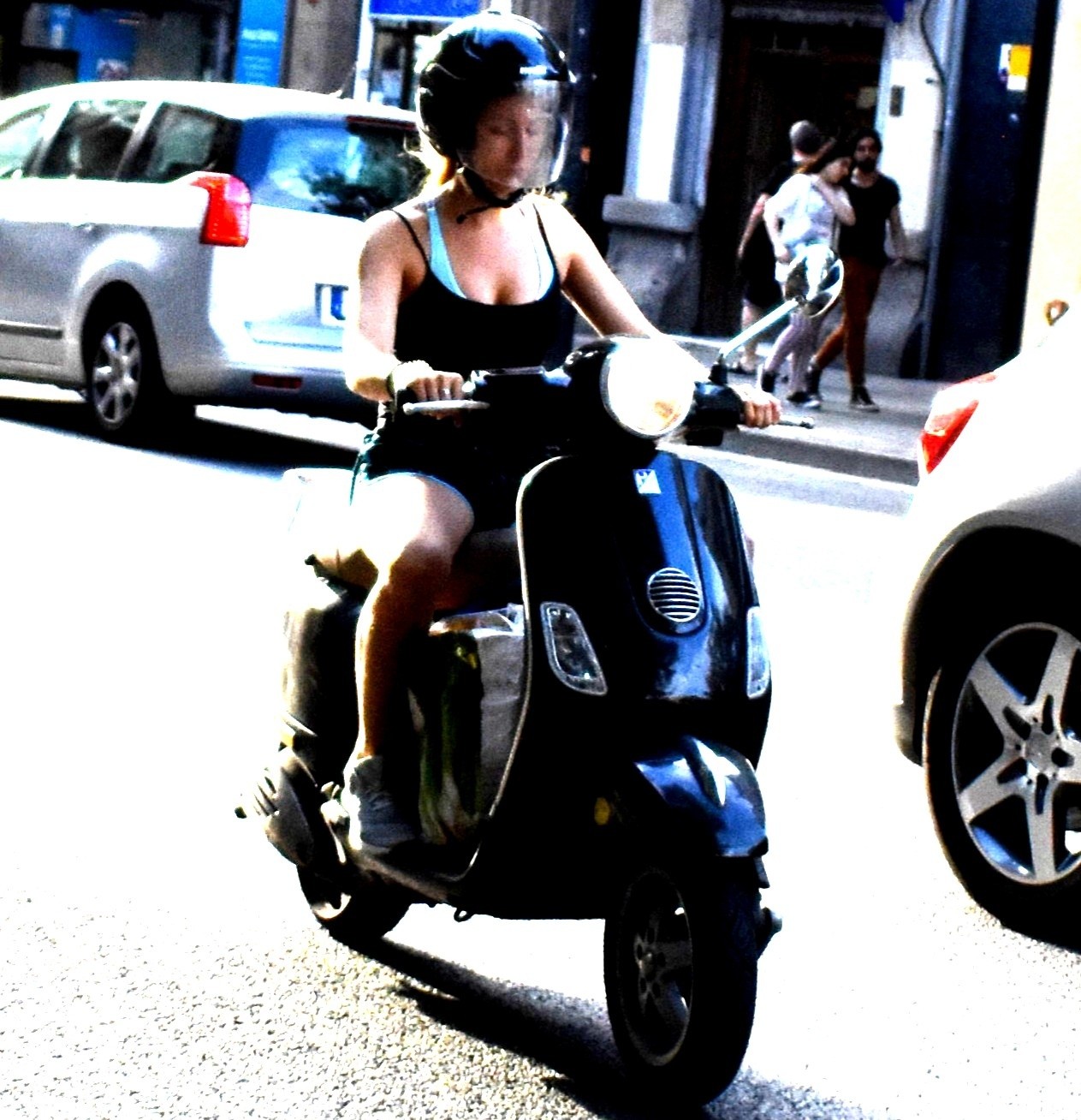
[231,240,848,1112]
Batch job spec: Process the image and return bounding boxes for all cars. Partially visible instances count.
[891,294,1081,941]
[0,76,449,448]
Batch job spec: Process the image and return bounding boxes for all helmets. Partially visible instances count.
[418,8,573,156]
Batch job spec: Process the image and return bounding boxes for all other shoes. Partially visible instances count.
[758,363,779,393]
[848,389,881,412]
[785,391,820,409]
[805,363,823,401]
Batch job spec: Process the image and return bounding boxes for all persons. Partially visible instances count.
[730,119,908,411]
[342,12,783,847]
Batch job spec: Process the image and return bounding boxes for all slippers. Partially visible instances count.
[724,359,750,374]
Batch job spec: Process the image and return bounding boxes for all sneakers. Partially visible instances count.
[337,743,417,852]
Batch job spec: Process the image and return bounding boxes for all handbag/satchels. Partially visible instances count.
[420,603,525,845]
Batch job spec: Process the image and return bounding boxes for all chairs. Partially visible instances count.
[66,123,131,178]
